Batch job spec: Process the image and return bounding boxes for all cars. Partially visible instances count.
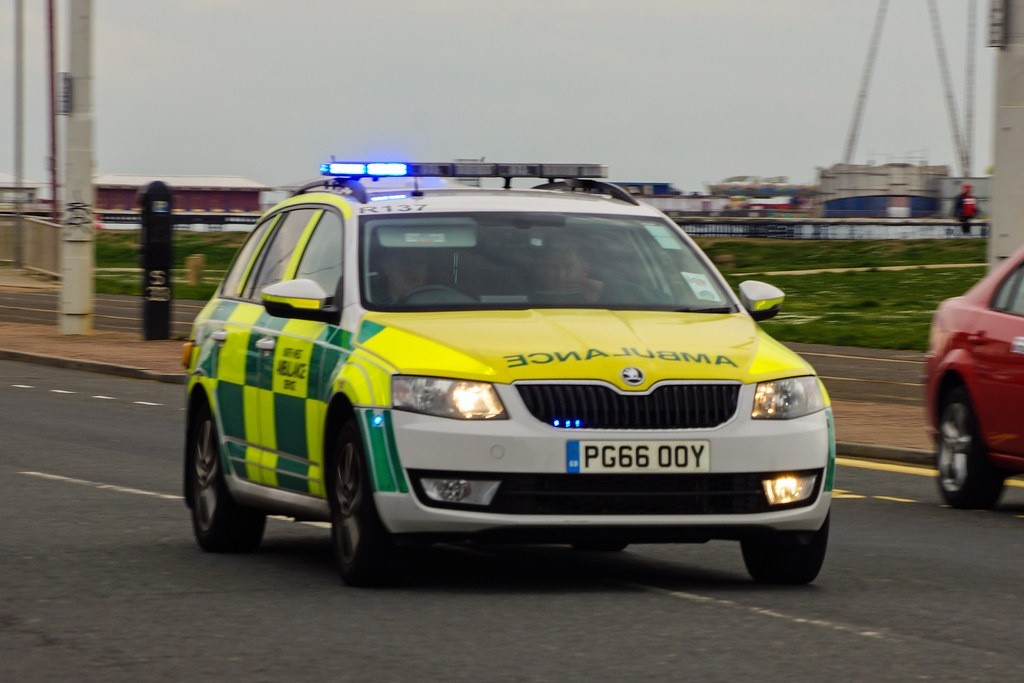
[925,246,1023,508]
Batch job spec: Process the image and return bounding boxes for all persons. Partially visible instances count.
[951,183,982,220]
[535,241,644,304]
[370,248,446,309]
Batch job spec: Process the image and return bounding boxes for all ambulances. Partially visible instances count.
[181,162,836,586]
